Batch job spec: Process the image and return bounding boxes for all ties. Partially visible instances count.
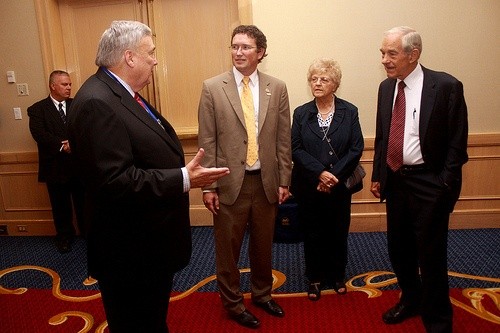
[386,80,407,172]
[59,103,66,126]
[135,94,164,128]
[241,76,259,168]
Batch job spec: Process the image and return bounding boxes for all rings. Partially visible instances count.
[330,183,332,185]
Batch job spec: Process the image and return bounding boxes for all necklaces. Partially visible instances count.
[316,94,334,115]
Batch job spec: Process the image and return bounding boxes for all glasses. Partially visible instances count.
[229,46,256,52]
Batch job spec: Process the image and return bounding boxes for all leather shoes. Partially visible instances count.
[59,239,71,253]
[237,310,260,327]
[260,299,285,317]
[382,302,417,323]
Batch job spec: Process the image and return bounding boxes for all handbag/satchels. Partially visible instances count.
[342,163,366,189]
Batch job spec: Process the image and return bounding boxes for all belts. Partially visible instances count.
[245,170,260,176]
[395,165,427,176]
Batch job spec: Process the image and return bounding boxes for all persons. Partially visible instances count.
[68,21,230,333]
[292,56,364,300]
[27,71,82,253]
[198,24,292,328]
[370,25,468,333]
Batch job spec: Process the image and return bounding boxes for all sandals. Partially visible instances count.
[333,278,346,294]
[308,280,321,300]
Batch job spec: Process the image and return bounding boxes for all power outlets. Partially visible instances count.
[16,225,28,232]
